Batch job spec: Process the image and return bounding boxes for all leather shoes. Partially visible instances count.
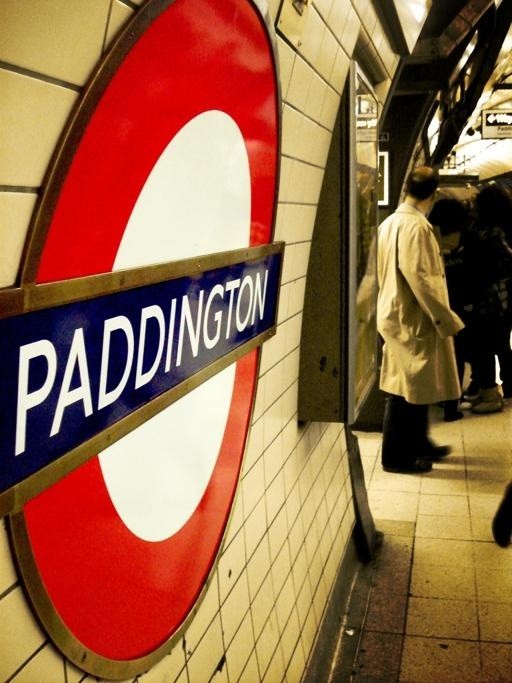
[420,439,452,460]
[382,457,434,475]
[444,411,464,422]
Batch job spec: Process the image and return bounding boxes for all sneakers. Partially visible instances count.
[492,483,512,548]
[460,384,504,414]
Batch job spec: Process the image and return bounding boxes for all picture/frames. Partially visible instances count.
[377,151,389,207]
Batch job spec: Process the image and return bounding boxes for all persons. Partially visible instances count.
[426,180,512,423]
[373,165,468,477]
